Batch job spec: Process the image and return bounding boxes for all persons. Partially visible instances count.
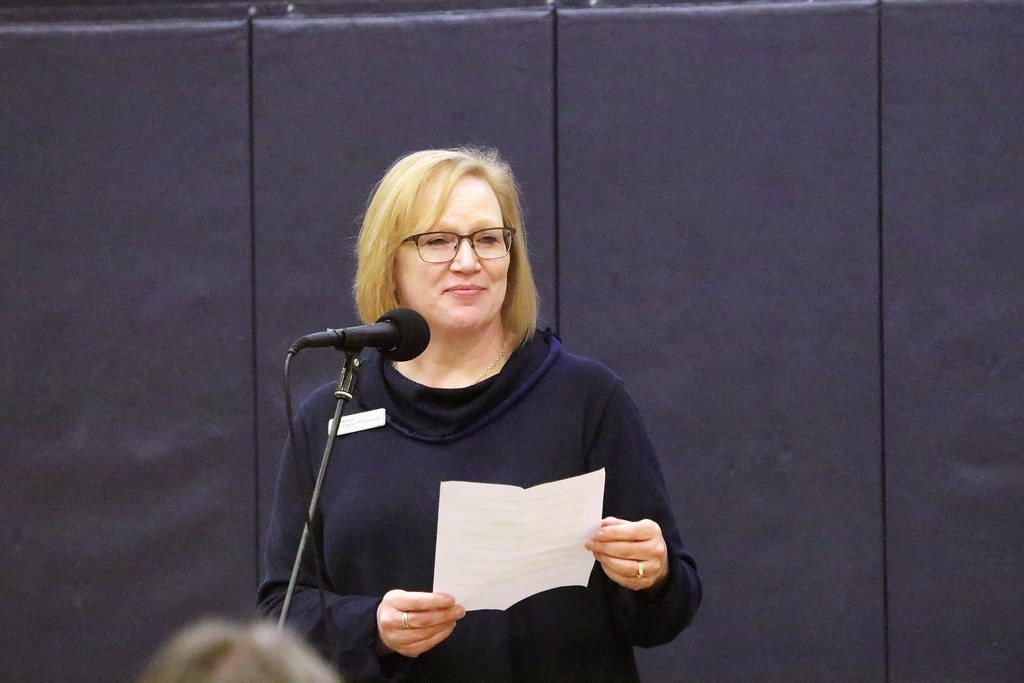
[253,150,703,683]
[143,617,342,683]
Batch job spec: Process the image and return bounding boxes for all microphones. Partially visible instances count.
[289,307,431,362]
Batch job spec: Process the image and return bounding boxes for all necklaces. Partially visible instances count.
[392,340,510,384]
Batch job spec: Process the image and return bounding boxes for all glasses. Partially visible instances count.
[401,227,516,263]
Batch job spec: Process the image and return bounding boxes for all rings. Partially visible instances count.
[401,612,409,628]
[637,563,644,578]
